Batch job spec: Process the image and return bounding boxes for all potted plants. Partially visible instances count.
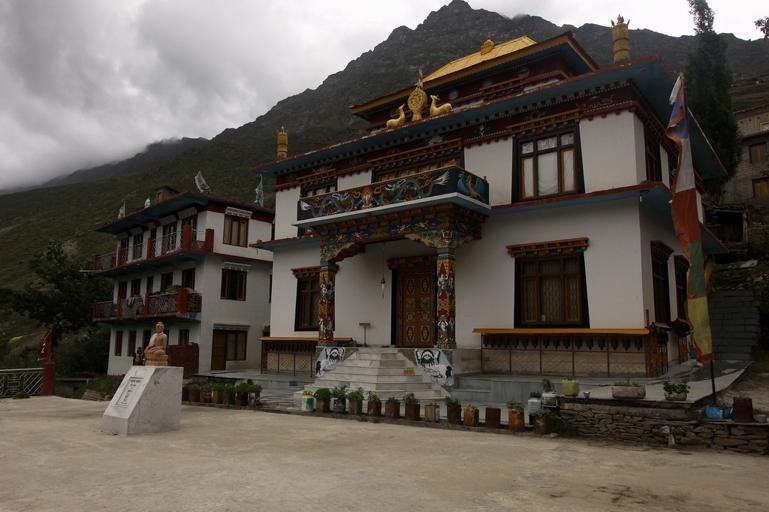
[661,378,691,400]
[611,373,646,399]
[561,370,580,397]
[182,378,263,407]
[314,378,557,434]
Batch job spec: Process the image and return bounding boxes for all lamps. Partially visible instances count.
[381,250,386,299]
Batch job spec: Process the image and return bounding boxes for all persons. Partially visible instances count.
[134,347,143,362]
[144,322,169,367]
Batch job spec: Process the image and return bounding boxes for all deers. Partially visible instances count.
[428,93,452,117]
[385,103,407,128]
